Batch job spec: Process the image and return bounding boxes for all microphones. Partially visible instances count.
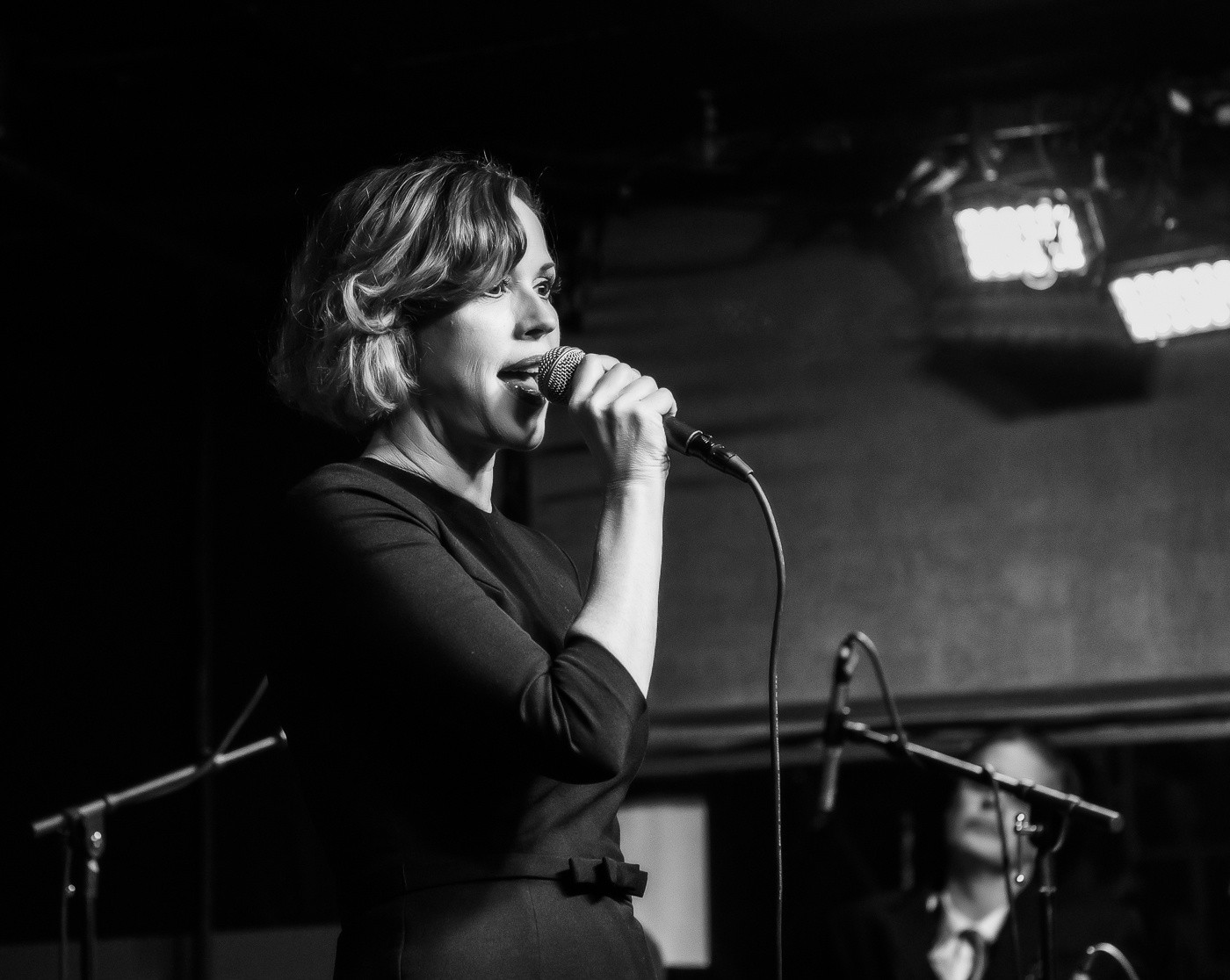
[538,346,755,482]
[816,641,855,813]
[1071,945,1096,980]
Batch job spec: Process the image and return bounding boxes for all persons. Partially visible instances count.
[873,736,1077,980]
[271,155,679,980]
